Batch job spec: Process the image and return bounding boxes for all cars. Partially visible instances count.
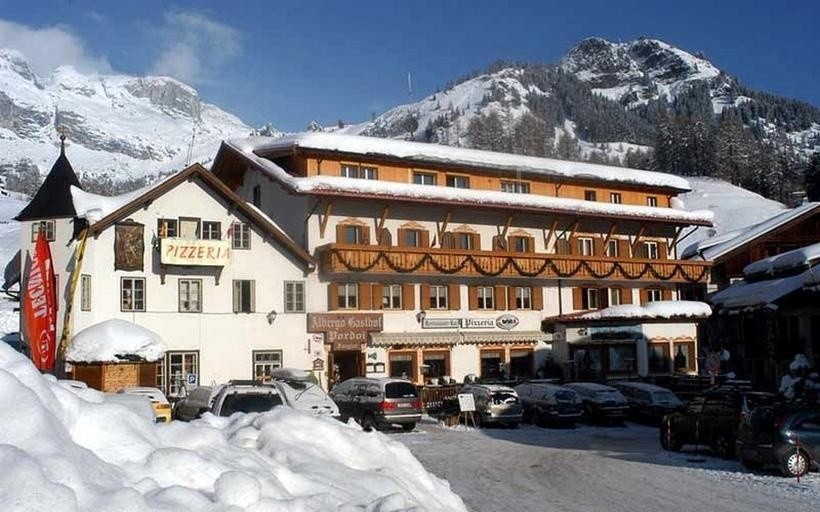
[512,383,584,426]
[115,386,172,424]
[612,382,684,418]
[456,384,523,425]
[732,400,820,480]
[561,384,634,424]
[267,368,341,419]
[658,386,781,452]
[173,379,287,424]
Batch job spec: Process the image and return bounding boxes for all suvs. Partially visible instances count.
[329,375,423,432]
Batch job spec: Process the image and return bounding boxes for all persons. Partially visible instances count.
[717,346,730,368]
[705,350,720,386]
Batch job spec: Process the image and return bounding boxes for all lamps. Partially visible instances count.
[267,310,277,320]
[418,310,426,320]
